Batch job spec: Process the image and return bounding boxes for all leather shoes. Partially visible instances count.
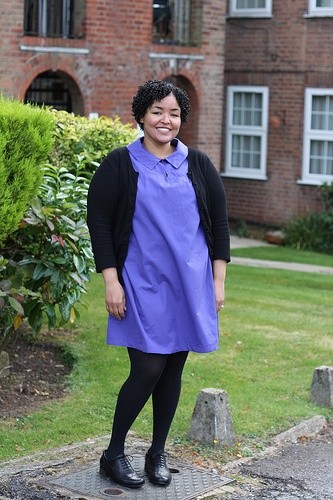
[143,449,172,485]
[99,450,145,487]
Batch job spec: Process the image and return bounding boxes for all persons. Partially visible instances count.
[86,77,232,488]
